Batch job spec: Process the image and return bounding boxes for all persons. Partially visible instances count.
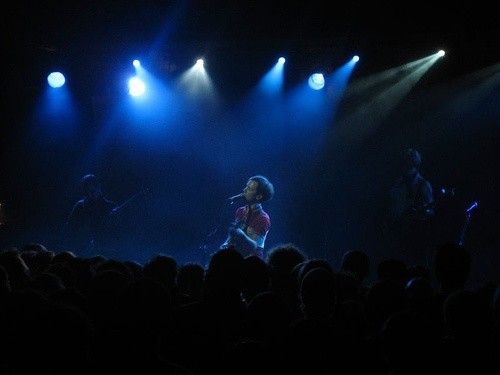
[0,241,500,375]
[219,175,273,261]
[384,144,435,265]
[59,171,125,262]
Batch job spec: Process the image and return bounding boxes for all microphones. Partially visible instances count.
[230,193,245,202]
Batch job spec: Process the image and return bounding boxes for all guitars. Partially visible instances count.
[221,222,246,250]
[70,188,153,255]
